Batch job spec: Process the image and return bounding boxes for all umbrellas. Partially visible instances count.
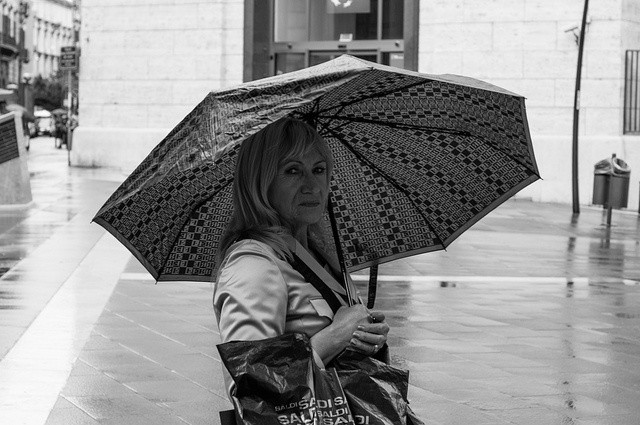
[89,53,542,308]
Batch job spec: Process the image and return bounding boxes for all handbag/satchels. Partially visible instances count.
[216,331,422,424]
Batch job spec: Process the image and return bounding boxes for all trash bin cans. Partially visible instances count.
[607,154,631,209]
[592,160,611,204]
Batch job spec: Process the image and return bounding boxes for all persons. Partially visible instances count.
[211,117,391,425]
